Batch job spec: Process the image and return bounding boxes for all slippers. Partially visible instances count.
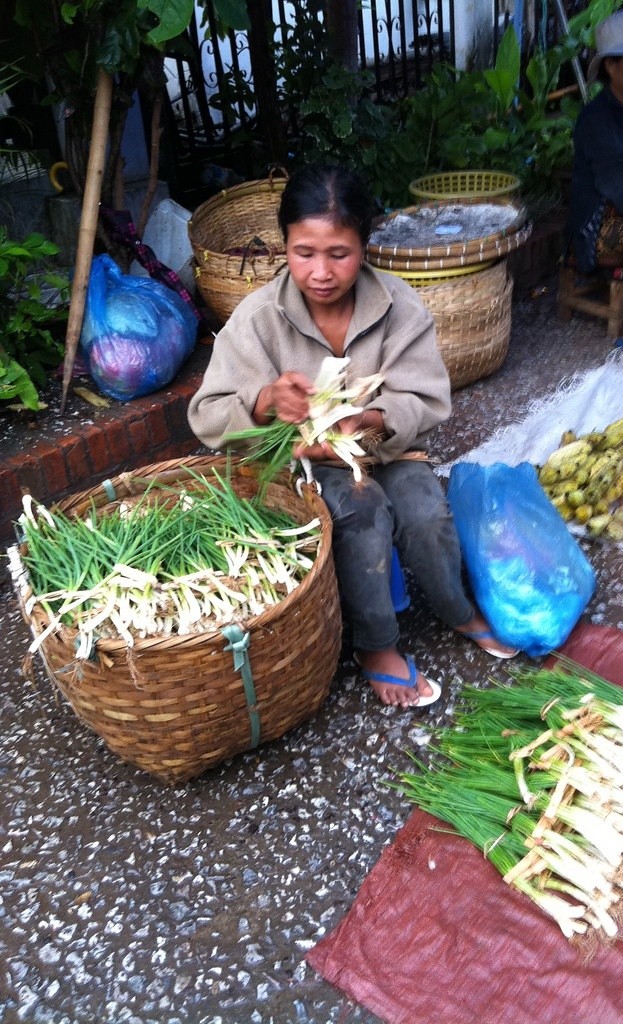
[355,652,448,715]
[442,610,522,661]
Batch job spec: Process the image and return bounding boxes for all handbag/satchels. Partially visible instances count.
[444,451,597,659]
[71,255,198,399]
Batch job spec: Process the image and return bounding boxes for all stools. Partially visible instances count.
[553,247,623,337]
[384,537,411,612]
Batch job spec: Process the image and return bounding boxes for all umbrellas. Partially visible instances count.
[50,159,219,341]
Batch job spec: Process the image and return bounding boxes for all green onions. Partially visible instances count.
[378,648,622,961]
[2,356,384,693]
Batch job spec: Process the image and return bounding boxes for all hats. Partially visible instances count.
[584,8,622,80]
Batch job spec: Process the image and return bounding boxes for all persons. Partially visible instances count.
[189,165,521,708]
[565,12,623,283]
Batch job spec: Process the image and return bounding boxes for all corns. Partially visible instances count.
[532,410,622,544]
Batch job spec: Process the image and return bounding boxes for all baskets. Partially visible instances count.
[189,167,302,332]
[14,455,338,785]
[372,195,533,267]
[376,262,514,401]
[405,167,519,205]
